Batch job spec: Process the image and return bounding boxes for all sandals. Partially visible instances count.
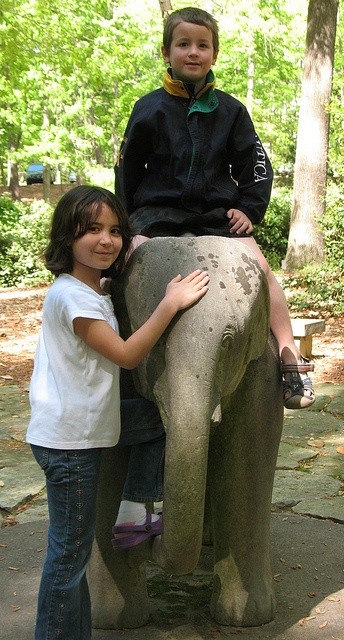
[283,355,315,409]
[109,506,166,555]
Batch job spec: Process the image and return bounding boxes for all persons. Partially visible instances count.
[114,6,315,409]
[26,185,208,640]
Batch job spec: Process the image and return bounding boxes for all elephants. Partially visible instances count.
[85,235,282,628]
